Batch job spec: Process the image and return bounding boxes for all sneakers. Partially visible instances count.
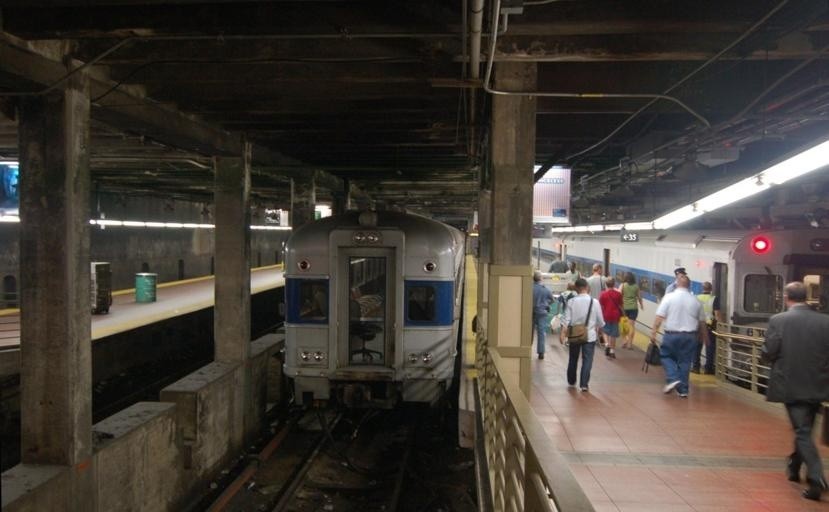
[609,353,616,358]
[663,380,682,394]
[674,388,688,397]
[580,384,588,391]
[605,344,610,356]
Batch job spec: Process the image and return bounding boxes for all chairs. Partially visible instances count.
[308,283,381,320]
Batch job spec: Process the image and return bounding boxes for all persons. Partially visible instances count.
[665,268,692,296]
[759,279,829,499]
[690,281,721,374]
[533,253,645,359]
[649,275,711,397]
[561,278,606,392]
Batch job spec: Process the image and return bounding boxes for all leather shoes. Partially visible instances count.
[803,475,828,500]
[786,452,801,482]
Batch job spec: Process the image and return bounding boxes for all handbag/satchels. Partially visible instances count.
[619,316,630,335]
[645,340,660,366]
[567,325,588,344]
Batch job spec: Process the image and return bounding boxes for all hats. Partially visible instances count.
[675,268,687,274]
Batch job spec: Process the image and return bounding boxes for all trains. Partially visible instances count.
[531,221,828,386]
[276,202,466,413]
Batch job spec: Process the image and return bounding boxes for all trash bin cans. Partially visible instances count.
[136,273,157,302]
